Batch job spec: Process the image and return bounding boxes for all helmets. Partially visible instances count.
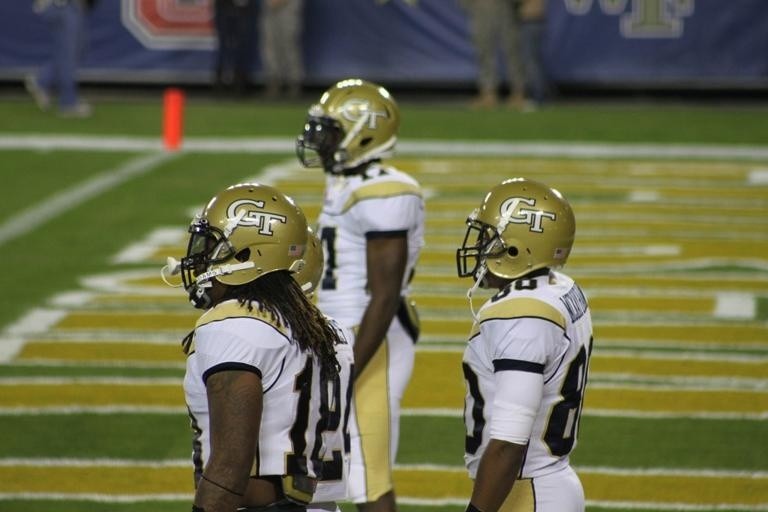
[181,182,307,309]
[296,78,400,172]
[290,227,325,298]
[456,176,576,289]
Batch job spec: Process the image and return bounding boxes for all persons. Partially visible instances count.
[175,181,357,510]
[292,77,428,511]
[512,0,551,113]
[210,0,265,97]
[18,0,99,121]
[455,177,599,511]
[283,223,356,512]
[255,1,307,103]
[464,1,527,112]
